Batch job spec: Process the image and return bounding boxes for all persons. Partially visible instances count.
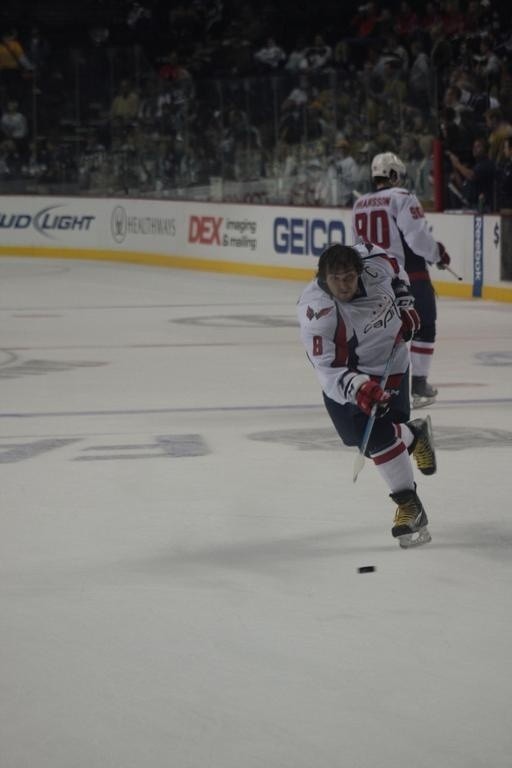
[2,2,510,215]
[350,148,454,412]
[295,241,438,538]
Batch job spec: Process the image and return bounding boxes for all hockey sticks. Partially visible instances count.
[354,324,402,481]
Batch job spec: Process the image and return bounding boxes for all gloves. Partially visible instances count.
[437,240,450,268]
[345,374,393,418]
[394,290,420,341]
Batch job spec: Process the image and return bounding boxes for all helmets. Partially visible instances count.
[370,152,406,185]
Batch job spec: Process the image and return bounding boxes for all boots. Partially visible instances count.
[405,418,435,475]
[390,481,428,537]
